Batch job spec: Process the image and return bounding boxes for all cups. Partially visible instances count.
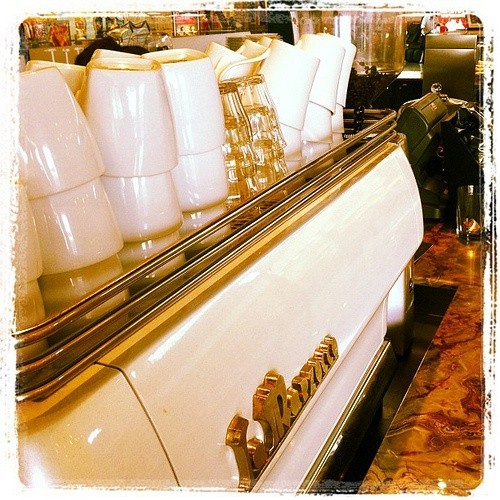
[18,31,358,282]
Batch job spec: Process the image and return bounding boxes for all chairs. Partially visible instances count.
[422,33,478,108]
